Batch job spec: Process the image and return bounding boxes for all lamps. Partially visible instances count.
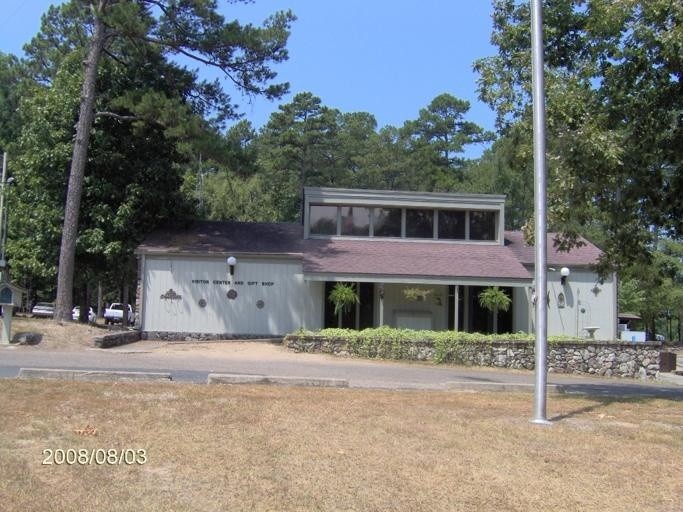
[225,255,238,277]
[559,264,570,283]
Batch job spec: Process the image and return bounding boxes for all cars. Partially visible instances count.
[71,306,96,324]
[31,302,56,318]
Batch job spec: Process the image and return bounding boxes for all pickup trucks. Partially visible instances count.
[104,302,136,327]
